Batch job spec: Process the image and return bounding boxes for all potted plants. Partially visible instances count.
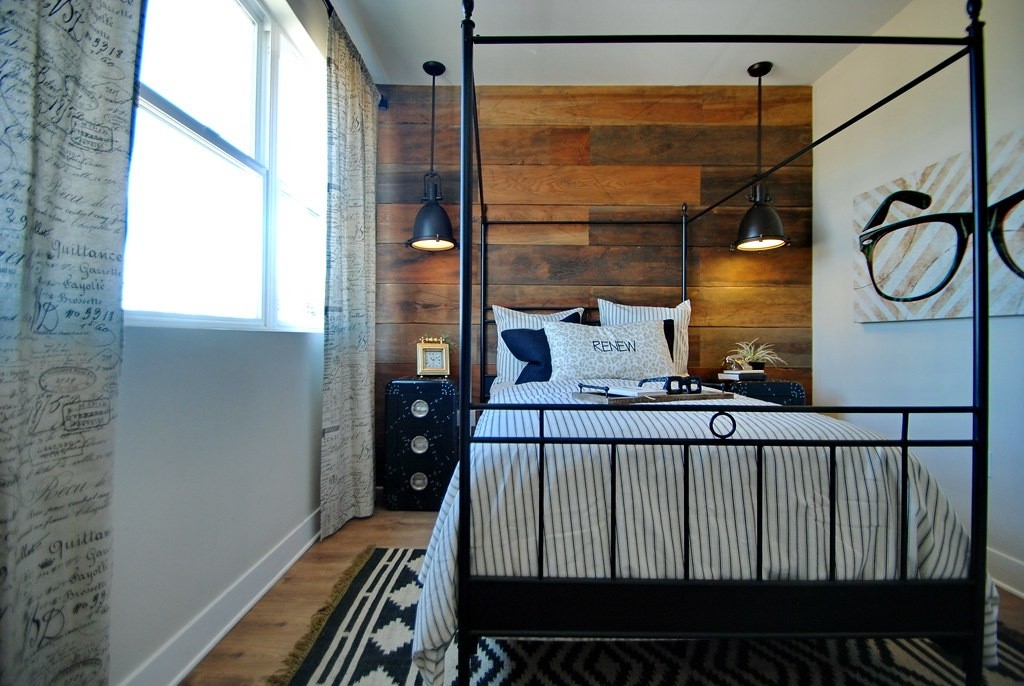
[720,336,789,370]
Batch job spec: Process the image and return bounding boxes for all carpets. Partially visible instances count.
[258,541,1023,686]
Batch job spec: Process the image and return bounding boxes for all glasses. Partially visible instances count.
[637,375,702,395]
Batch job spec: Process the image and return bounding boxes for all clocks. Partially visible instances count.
[417,337,450,378]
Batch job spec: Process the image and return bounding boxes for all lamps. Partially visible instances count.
[404,60,460,253]
[729,61,792,253]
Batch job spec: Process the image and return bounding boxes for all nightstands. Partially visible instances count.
[383,376,458,512]
[709,381,806,408]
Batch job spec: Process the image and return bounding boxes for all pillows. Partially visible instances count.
[491,304,585,385]
[501,312,581,385]
[597,298,692,378]
[542,319,684,383]
[661,319,673,364]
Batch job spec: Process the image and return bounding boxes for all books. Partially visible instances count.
[604,387,668,396]
[718,369,767,382]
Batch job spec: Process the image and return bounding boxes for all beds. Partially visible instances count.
[411,1,1002,686]
